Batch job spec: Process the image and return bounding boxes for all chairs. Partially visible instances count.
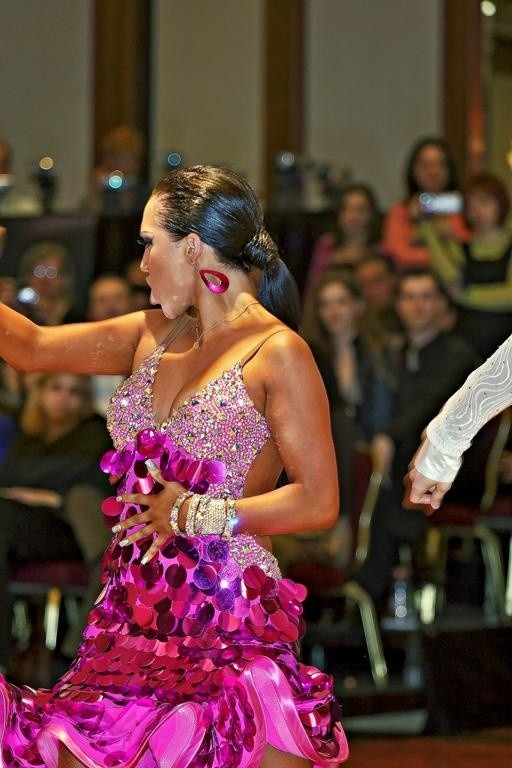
[2,409,511,707]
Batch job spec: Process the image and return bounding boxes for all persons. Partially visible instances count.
[290,132,512,692]
[0,160,354,768]
[2,243,152,592]
